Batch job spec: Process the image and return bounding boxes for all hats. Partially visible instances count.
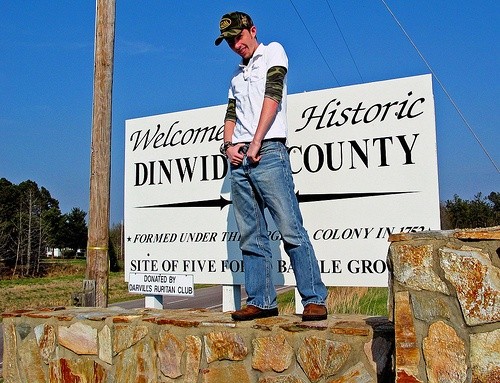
[215,11,253,46]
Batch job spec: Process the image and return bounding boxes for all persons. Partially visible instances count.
[214,11,329,321]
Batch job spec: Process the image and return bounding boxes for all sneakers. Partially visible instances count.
[302,304,327,320]
[231,306,278,321]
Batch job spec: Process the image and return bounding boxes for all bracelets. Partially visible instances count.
[224,140,232,150]
